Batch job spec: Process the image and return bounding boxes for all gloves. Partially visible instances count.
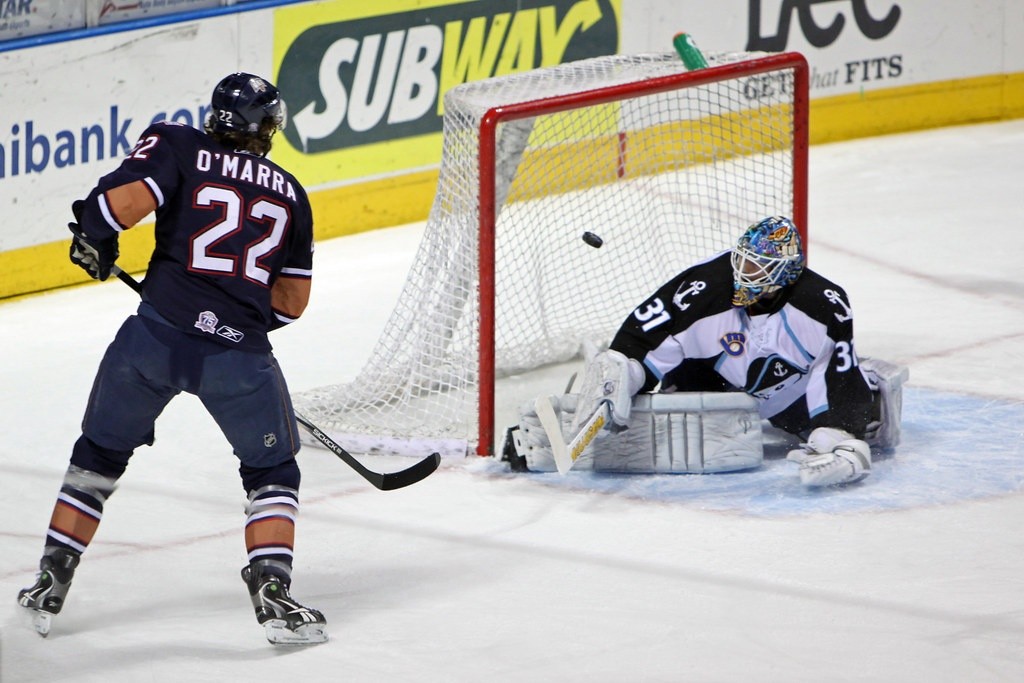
[573,349,633,435]
[69,231,121,282]
[785,427,873,487]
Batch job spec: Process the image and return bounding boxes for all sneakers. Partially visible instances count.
[240,563,327,643]
[17,545,81,635]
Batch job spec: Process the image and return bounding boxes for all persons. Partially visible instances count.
[516,215,910,490]
[16,73,329,645]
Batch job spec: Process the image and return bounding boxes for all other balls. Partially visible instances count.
[582,230,605,249]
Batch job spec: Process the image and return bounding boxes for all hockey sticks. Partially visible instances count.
[65,220,442,493]
[533,393,611,476]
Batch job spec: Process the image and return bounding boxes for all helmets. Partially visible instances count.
[730,215,804,307]
[209,72,284,134]
